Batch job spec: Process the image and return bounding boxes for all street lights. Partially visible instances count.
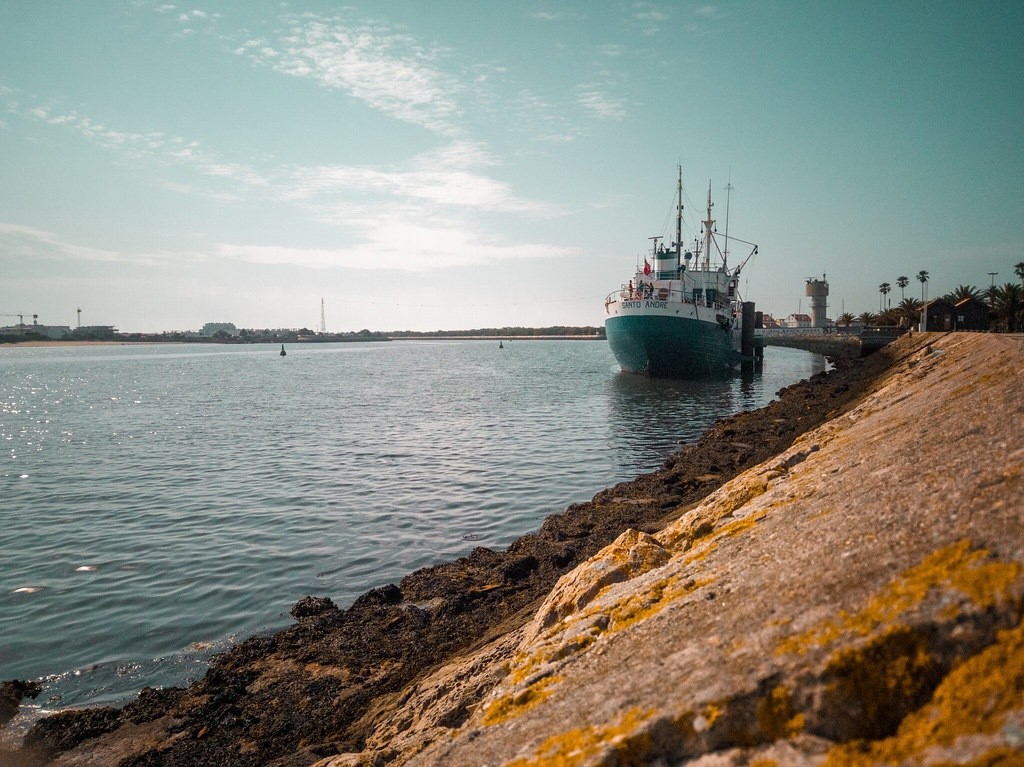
[988,272,999,287]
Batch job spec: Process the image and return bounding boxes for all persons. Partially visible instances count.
[639,280,644,292]
[900,317,913,327]
[647,283,654,299]
[629,280,633,300]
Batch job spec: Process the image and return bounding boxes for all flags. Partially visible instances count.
[644,259,650,276]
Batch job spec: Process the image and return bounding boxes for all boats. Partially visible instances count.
[604,166,758,378]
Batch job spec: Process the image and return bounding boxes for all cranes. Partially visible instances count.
[0,314,38,326]
